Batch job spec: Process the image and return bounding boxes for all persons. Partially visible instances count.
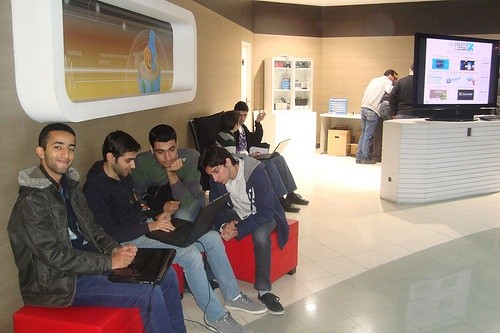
[7,124,187,333]
[356,70,399,164]
[83,131,268,333]
[234,101,310,212]
[201,147,289,316]
[389,64,425,119]
[201,110,240,191]
[129,124,227,293]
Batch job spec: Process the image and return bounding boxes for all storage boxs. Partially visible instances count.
[327,127,360,157]
[328,98,348,115]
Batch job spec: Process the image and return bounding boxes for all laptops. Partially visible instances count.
[145,191,230,247]
[249,138,291,159]
[108,248,177,285]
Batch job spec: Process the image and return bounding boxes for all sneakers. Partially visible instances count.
[258,291,285,315]
[286,193,309,205]
[279,196,301,212]
[211,311,253,333]
[224,292,268,314]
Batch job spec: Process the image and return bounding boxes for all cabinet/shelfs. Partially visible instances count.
[263,58,313,112]
[254,109,317,154]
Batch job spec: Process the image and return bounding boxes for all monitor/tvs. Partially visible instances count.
[328,98,348,115]
[413,32,500,122]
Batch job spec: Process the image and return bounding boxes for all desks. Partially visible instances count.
[321,112,361,153]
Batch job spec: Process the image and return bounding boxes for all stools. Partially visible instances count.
[12,262,184,333]
[223,219,298,291]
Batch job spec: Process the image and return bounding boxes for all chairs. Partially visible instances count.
[189,112,226,191]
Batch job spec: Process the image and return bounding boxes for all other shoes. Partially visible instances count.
[356,158,377,164]
